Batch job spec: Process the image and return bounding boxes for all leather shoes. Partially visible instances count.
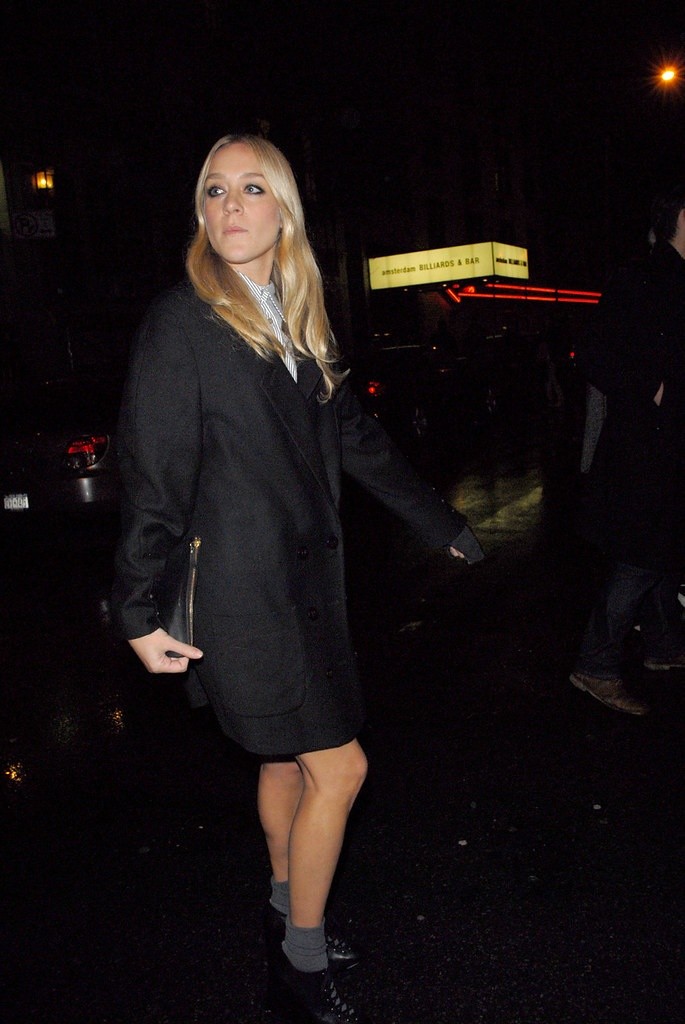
[265,943,364,1024]
[570,671,652,717]
[643,646,685,670]
[261,899,368,970]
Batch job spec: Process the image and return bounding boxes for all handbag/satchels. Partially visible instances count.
[91,535,202,658]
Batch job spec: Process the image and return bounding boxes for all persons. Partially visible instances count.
[112,135,485,1024]
[571,188,685,716]
